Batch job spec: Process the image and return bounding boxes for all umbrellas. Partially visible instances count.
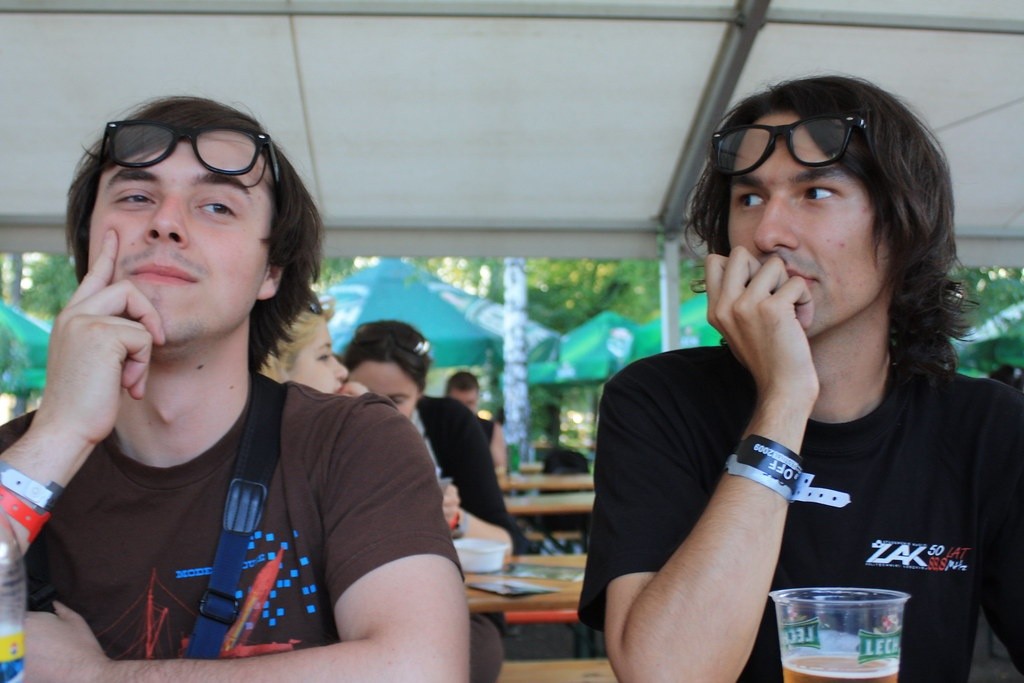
[952,298,1024,376]
[640,290,723,356]
[529,310,661,467]
[320,257,554,368]
[0,300,50,397]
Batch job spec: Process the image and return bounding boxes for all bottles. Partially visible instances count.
[0,504,32,683]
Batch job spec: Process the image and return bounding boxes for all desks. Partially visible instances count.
[448,463,621,683]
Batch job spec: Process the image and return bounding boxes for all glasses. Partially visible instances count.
[299,290,321,314]
[712,113,867,175]
[355,322,429,355]
[99,119,279,182]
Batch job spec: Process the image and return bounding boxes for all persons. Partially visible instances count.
[257,287,366,397]
[0,96,470,683]
[343,321,515,683]
[579,77,1024,683]
[445,373,510,484]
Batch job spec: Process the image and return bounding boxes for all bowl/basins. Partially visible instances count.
[453,538,510,573]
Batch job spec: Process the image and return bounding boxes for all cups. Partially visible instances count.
[769,587,912,683]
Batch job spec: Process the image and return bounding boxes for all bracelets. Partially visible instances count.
[449,510,468,534]
[723,436,851,508]
[0,461,61,508]
[0,488,51,543]
